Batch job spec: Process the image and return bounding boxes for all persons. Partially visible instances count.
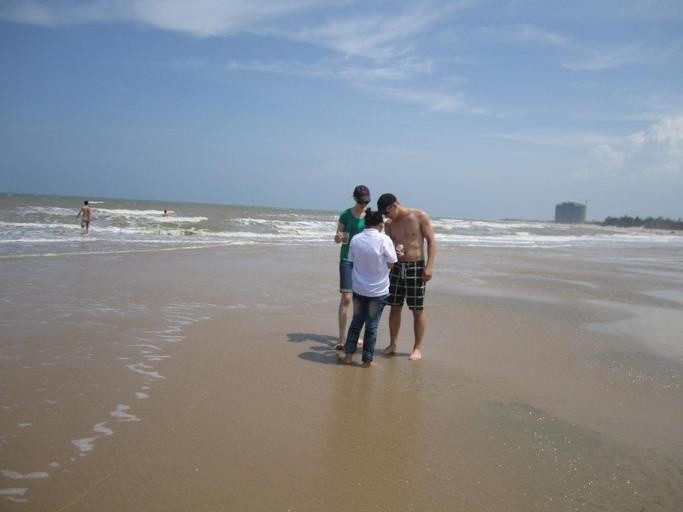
[334,185,437,368]
[76,201,92,234]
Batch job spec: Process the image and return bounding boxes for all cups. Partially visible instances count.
[396,245,404,256]
[340,232,349,245]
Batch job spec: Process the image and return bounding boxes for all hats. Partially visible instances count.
[353,195,371,202]
